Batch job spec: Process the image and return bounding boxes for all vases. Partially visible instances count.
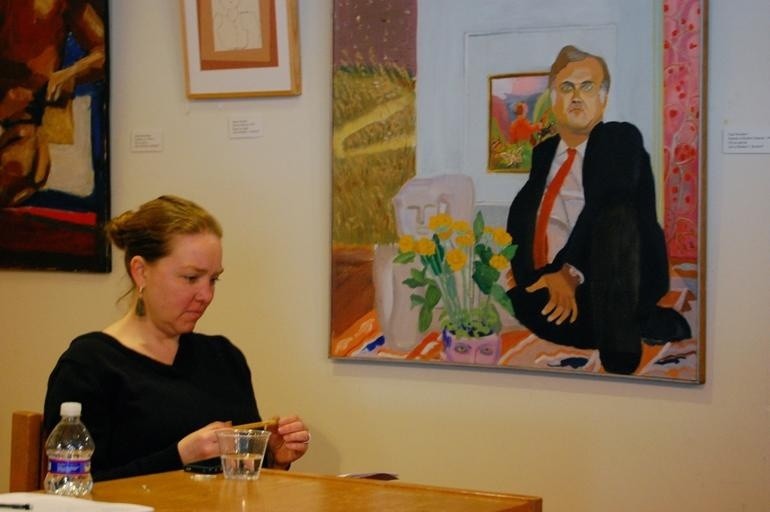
[443,326,501,365]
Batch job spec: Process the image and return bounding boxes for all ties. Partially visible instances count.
[534,148,577,271]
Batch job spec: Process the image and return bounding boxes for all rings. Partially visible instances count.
[306,432,312,444]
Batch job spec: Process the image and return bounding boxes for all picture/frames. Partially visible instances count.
[0,0,111,273]
[179,0,301,99]
[486,71,556,173]
[327,1,709,385]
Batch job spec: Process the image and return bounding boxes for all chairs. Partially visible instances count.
[9,410,47,492]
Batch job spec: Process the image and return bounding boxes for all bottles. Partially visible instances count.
[43,401,95,497]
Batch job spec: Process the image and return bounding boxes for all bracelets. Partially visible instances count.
[565,265,582,282]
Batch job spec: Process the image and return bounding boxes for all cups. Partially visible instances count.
[215,429,271,480]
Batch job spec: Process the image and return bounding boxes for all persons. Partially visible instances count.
[0,1,107,195]
[45,194,312,483]
[504,44,692,375]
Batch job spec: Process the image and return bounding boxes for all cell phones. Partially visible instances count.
[184,456,228,474]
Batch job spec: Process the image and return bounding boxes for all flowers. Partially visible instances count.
[393,210,519,340]
